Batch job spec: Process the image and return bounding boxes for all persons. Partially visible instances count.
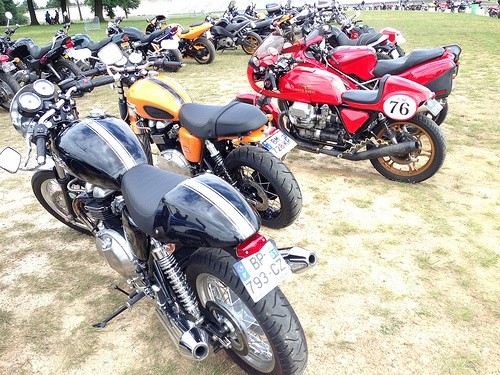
[446,0,454,10]
[45,11,50,25]
[362,1,364,5]
[434,0,441,11]
[458,0,500,13]
[381,1,424,11]
[55,10,59,24]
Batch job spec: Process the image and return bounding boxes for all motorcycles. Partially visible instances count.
[0,0,461,126]
[228,27,446,184]
[0,71,316,375]
[78,40,303,230]
